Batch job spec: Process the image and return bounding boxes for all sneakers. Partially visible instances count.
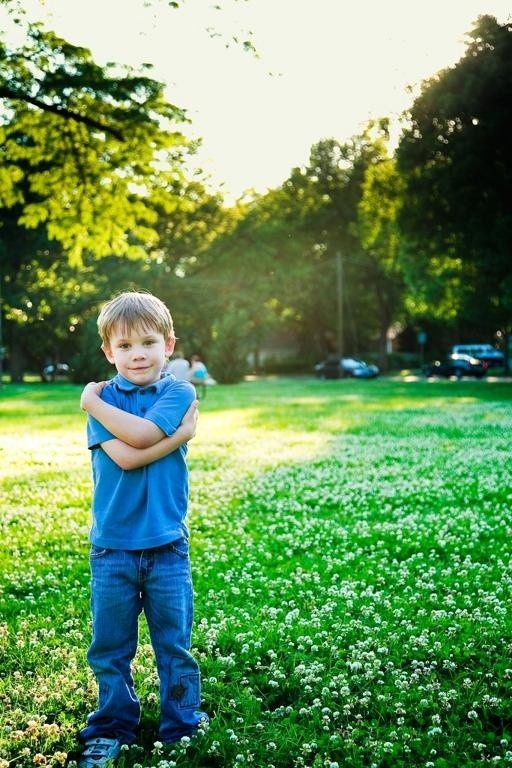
[78,738,121,768]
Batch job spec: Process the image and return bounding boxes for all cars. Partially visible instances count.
[424,344,505,377]
[314,353,380,379]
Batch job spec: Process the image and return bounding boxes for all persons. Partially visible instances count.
[77,290,200,768]
[162,350,210,384]
